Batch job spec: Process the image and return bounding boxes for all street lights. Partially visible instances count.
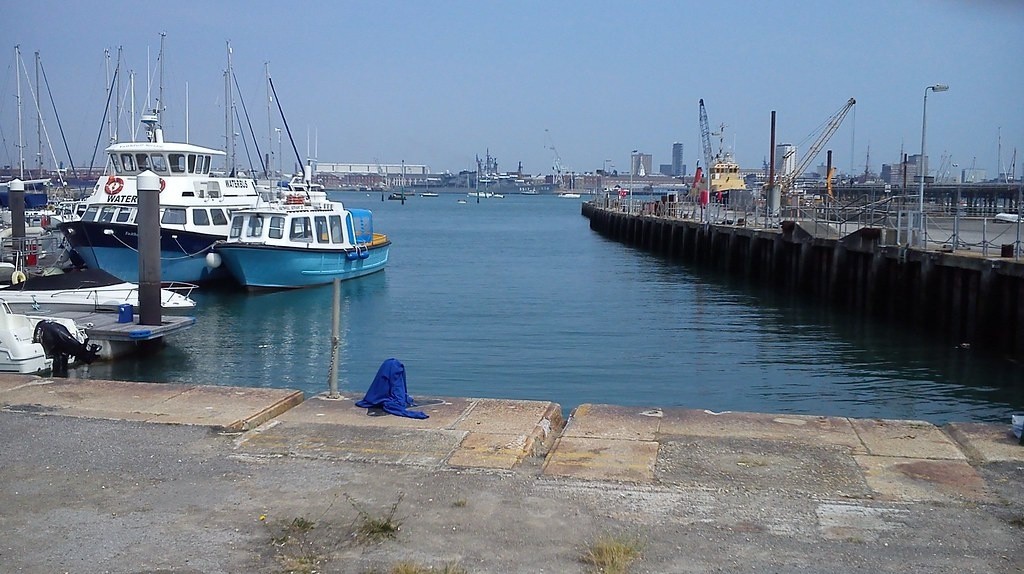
[915,85,949,245]
[629,149,638,213]
[602,159,611,208]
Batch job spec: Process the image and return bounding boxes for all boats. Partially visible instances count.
[457,200,467,204]
[468,192,505,199]
[0,299,103,374]
[388,194,406,200]
[420,193,438,197]
[393,192,415,196]
[558,194,580,198]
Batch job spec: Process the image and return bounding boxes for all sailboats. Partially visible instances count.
[213,62,391,287]
[1,28,342,284]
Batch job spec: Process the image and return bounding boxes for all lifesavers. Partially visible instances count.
[104,179,124,194]
[160,177,165,192]
[41,216,51,227]
